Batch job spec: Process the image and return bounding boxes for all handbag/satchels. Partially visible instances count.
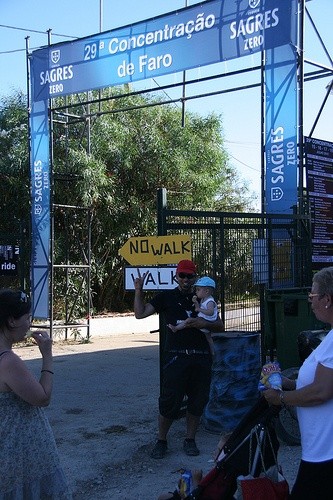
[234,422,291,500]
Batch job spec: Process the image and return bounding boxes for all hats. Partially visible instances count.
[176,259,196,274]
[194,276,216,289]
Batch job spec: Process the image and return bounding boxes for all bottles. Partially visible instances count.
[266,349,271,363]
[273,349,278,363]
[179,466,192,497]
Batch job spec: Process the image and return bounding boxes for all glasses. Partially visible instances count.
[178,273,193,280]
[11,289,28,316]
[307,291,325,303]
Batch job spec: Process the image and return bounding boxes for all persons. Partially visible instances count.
[132,260,224,459]
[167,276,219,357]
[260,267,333,500]
[0,290,73,500]
[157,430,233,500]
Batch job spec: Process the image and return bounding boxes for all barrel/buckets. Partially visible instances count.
[202,330,261,436]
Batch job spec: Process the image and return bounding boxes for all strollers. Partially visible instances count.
[167,395,291,500]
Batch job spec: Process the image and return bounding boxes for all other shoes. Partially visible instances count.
[151,440,168,459]
[183,439,200,455]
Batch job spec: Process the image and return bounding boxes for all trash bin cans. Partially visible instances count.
[296,330,328,366]
[263,287,330,368]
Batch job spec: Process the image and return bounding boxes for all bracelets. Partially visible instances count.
[280,391,287,407]
[135,292,143,296]
[41,369,54,374]
